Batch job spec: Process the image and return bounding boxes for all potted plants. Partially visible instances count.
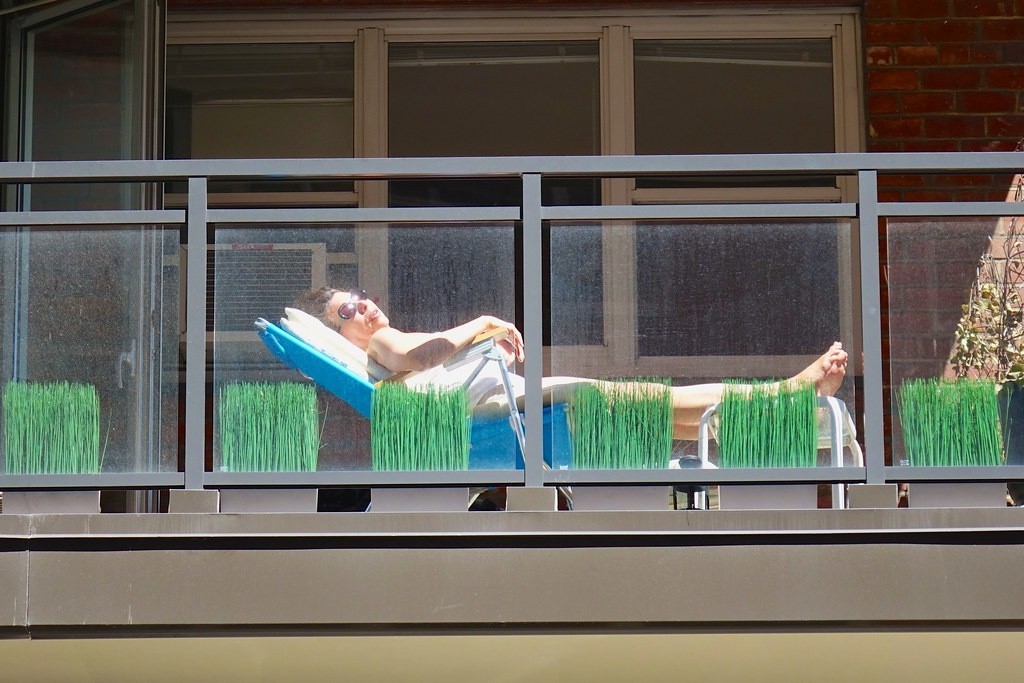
[719,376,818,509]
[564,375,672,511]
[2,380,113,515]
[218,379,329,512]
[896,378,1007,508]
[368,382,470,511]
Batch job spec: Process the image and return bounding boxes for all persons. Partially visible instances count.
[296,285,849,440]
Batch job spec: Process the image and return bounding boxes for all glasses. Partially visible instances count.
[337,289,367,333]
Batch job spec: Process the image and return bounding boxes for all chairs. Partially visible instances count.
[255,306,863,511]
